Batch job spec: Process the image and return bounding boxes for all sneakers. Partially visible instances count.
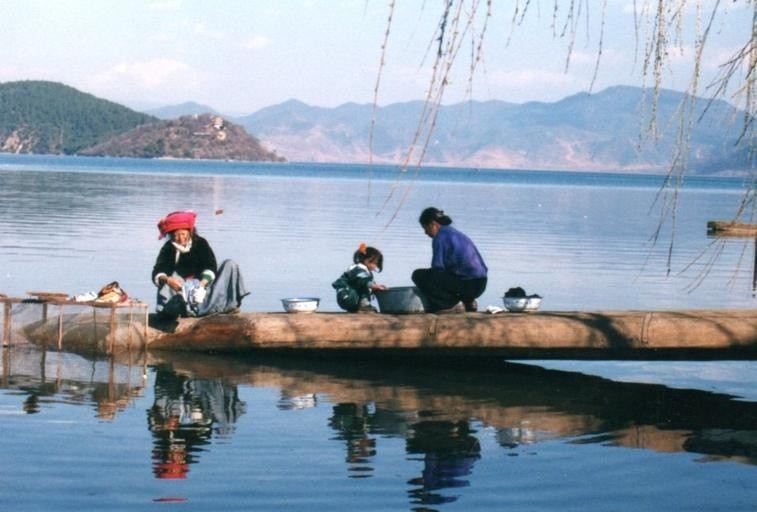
[465,301,478,311]
[357,304,377,313]
[436,302,465,315]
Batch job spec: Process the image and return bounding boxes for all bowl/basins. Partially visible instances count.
[501,297,543,312]
[372,286,424,312]
[281,298,320,312]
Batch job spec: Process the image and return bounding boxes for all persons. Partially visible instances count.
[332,243,383,312]
[152,211,250,317]
[147,367,237,478]
[327,404,376,462]
[411,207,487,312]
[404,420,480,512]
